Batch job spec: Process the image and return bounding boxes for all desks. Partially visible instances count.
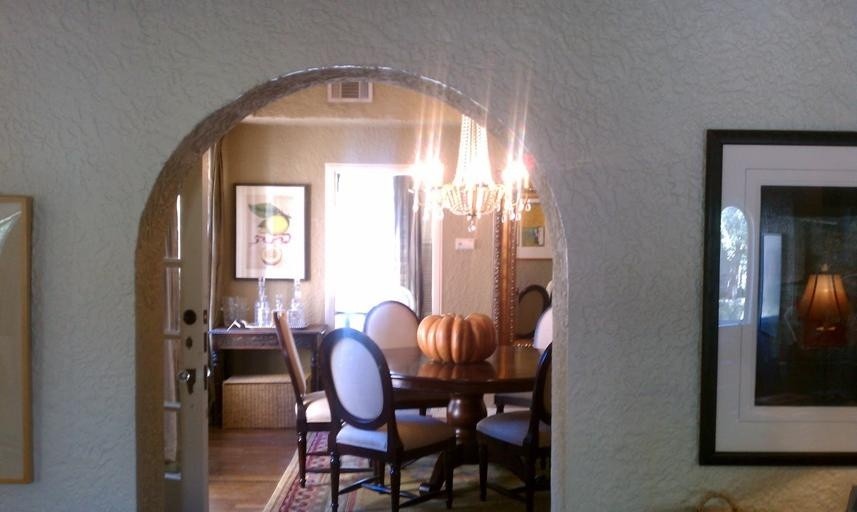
[209,324,326,431]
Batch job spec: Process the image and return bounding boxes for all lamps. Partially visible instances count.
[431,114,503,233]
[796,262,852,402]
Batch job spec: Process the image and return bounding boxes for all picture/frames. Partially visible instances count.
[704,127,856,469]
[233,182,312,282]
[0,195,33,486]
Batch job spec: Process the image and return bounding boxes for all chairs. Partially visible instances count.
[475,341,551,511]
[364,302,428,424]
[515,285,551,338]
[273,310,380,488]
[318,328,457,512]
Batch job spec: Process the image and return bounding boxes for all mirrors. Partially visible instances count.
[323,162,442,335]
[492,171,555,350]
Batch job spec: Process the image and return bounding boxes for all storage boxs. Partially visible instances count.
[221,373,310,429]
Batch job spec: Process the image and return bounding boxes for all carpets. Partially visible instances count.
[259,429,551,511]
